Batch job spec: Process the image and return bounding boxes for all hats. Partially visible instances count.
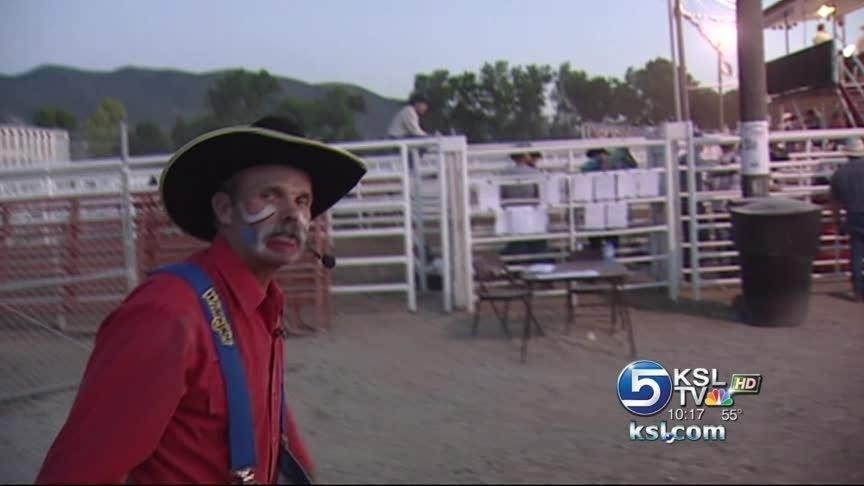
[159,116,367,241]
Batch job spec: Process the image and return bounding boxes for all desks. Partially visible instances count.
[518,260,636,365]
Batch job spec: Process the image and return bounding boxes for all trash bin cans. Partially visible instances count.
[727,195,822,327]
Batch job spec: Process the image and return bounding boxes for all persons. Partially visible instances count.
[32,114,368,486]
[387,93,430,139]
[830,136,864,302]
[496,142,548,253]
[586,148,626,170]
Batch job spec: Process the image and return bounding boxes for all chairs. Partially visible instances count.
[467,254,544,337]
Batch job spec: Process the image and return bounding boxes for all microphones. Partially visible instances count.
[305,242,336,269]
